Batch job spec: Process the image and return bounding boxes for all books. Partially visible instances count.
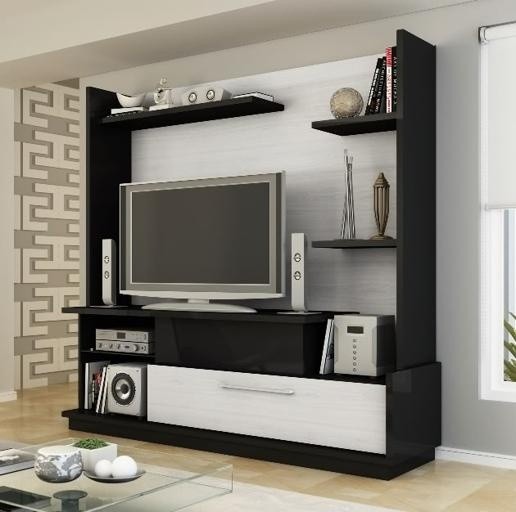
[319,318,335,375]
[364,45,397,116]
[84,361,112,415]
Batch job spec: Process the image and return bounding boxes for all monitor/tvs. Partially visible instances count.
[119,170,286,314]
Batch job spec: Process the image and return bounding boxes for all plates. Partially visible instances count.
[83,469,146,483]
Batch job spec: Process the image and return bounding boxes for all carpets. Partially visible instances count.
[0,440,405,512]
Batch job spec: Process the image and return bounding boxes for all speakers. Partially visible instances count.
[290,230,308,311]
[99,237,117,308]
[334,313,398,377]
[107,363,146,419]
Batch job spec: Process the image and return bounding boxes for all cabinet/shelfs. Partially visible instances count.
[62,28,442,482]
